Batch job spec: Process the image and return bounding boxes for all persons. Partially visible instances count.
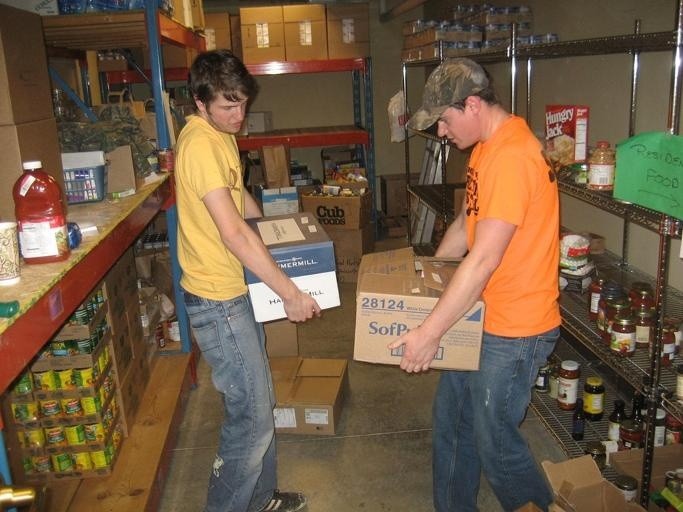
[385,55,563,511]
[171,45,324,512]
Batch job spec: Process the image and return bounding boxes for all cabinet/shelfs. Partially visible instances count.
[400,2,683,512]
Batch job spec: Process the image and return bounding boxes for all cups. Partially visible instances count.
[0,222,21,287]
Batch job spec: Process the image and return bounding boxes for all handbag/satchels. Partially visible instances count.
[103,88,157,140]
[388,90,417,143]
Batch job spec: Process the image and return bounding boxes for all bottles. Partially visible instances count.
[583,439,683,503]
[607,400,628,443]
[629,398,643,426]
[586,141,616,192]
[11,159,71,265]
[572,398,586,441]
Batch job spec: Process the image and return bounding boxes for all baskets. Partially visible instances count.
[62,158,112,205]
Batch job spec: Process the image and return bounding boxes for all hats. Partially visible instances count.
[407,57,490,131]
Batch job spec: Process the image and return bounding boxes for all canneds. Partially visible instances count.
[641,407,683,448]
[402,3,558,49]
[12,287,122,477]
[587,277,681,366]
[582,377,606,420]
[613,475,638,503]
[557,360,579,411]
[619,418,642,451]
[158,148,173,172]
[583,441,606,472]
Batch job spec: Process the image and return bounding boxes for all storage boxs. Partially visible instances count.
[352,246,486,373]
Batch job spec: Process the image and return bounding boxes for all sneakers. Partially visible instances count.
[259,492,307,512]
